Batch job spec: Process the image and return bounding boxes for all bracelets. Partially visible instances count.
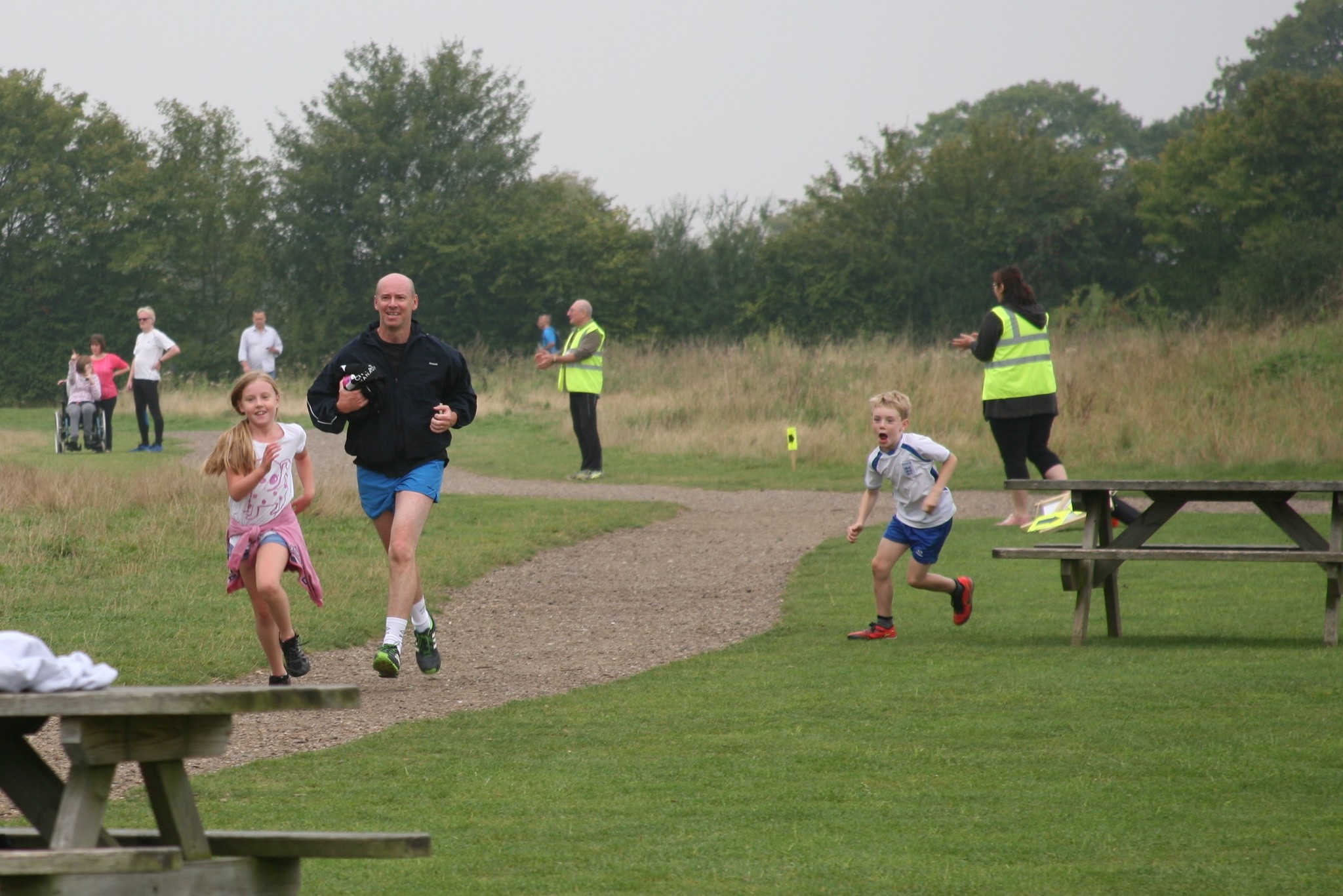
[553,356,556,363]
[159,359,163,363]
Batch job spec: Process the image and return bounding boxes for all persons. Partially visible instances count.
[306,273,478,679]
[846,392,975,640]
[535,298,606,479]
[57,306,181,451]
[202,370,316,686]
[239,308,282,383]
[951,266,1068,525]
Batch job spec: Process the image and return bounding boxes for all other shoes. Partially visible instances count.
[93,450,97,453]
[70,437,77,446]
[85,440,95,446]
[564,469,584,481]
[576,470,604,480]
[129,444,150,452]
[105,449,110,453]
[148,443,162,452]
[995,514,1032,526]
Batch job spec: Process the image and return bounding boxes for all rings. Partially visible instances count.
[441,426,443,429]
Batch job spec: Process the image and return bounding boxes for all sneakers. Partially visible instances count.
[414,611,441,675]
[951,576,974,625]
[279,630,310,677]
[373,641,401,678]
[269,671,293,686]
[847,622,897,640]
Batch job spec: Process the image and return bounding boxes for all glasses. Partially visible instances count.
[138,318,152,322]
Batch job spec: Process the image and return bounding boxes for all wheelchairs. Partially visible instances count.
[54,379,107,453]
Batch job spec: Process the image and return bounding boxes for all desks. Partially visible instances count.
[0,684,360,896]
[1004,478,1343,648]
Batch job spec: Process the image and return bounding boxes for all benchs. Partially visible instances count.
[0,827,433,896]
[992,544,1343,647]
